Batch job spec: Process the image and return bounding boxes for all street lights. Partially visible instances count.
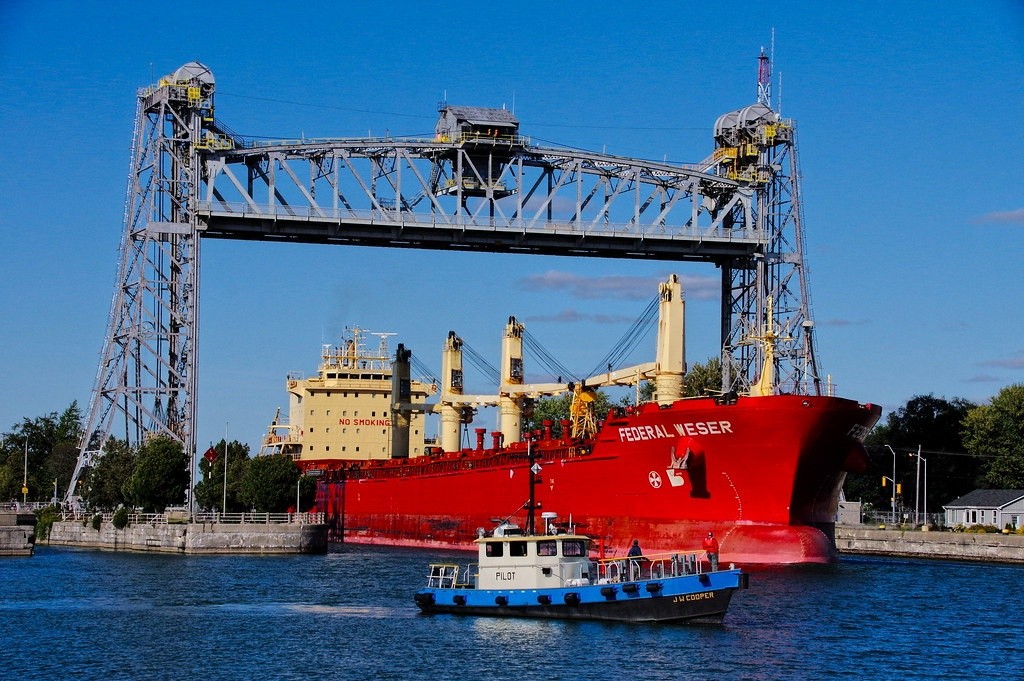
[23,435,29,504]
[223,421,230,517]
[297,477,301,513]
[909,453,927,526]
[884,444,896,523]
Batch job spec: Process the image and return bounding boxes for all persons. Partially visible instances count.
[626,539,651,580]
[9,497,18,513]
[703,531,719,568]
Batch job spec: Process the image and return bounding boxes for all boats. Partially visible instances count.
[259,322,472,521]
[413,446,749,629]
[311,296,882,577]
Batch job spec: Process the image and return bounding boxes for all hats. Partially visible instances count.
[708,532,713,536]
